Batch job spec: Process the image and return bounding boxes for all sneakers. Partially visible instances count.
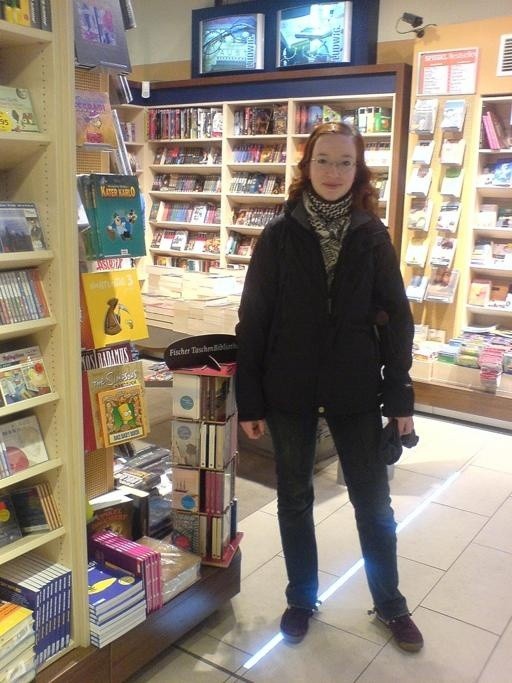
[279,605,314,644]
[375,608,424,652]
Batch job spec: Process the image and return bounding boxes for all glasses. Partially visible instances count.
[310,157,359,171]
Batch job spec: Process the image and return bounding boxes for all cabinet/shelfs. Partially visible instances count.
[0,0,90,683]
[110,93,396,273]
[461,95,512,333]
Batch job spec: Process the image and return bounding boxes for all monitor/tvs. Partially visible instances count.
[191,0,270,78]
[269,0,378,72]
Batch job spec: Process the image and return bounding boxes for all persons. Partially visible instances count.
[30,219,43,240]
[228,119,426,656]
[16,88,28,99]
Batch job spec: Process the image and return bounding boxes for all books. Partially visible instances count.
[297,104,392,135]
[1,0,52,32]
[74,89,118,151]
[1,417,64,546]
[171,371,237,561]
[365,141,391,219]
[81,269,149,452]
[86,485,203,649]
[2,268,51,327]
[147,108,222,274]
[244,103,287,259]
[76,173,146,261]
[2,551,72,682]
[72,0,133,78]
[404,97,512,311]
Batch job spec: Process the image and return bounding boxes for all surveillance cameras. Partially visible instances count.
[401,10,424,28]
